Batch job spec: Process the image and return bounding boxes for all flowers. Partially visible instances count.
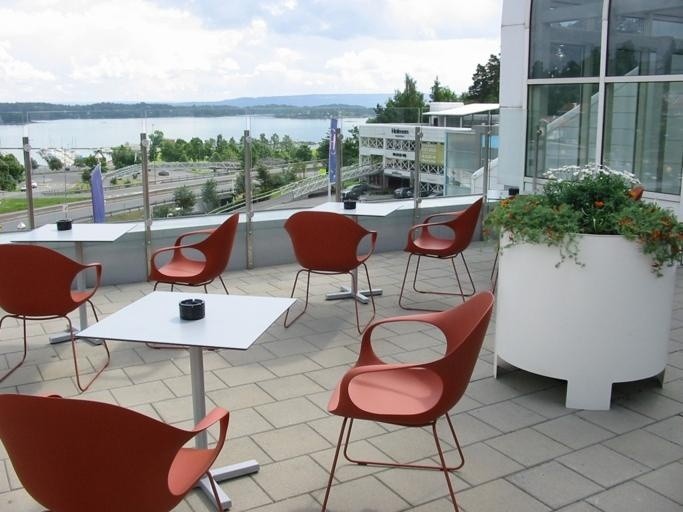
[482,171,683,280]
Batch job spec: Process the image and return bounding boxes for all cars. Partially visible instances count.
[157,169,171,177]
[393,187,410,199]
[19,180,37,193]
[338,183,368,202]
